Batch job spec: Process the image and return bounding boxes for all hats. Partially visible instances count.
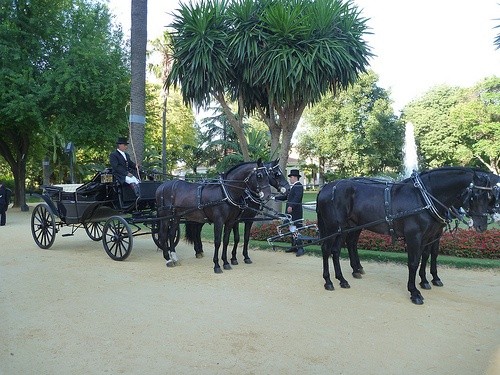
[287,169,301,177]
[116,138,129,144]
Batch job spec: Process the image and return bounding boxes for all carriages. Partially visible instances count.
[30,142,500,305]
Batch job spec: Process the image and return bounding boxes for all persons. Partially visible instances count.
[0,184,8,226]
[270,170,304,256]
[110,137,144,204]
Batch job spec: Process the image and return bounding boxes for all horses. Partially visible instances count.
[156,157,291,274]
[316,167,500,305]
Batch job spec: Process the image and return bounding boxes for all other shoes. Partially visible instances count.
[285,248,298,253]
[296,248,304,257]
